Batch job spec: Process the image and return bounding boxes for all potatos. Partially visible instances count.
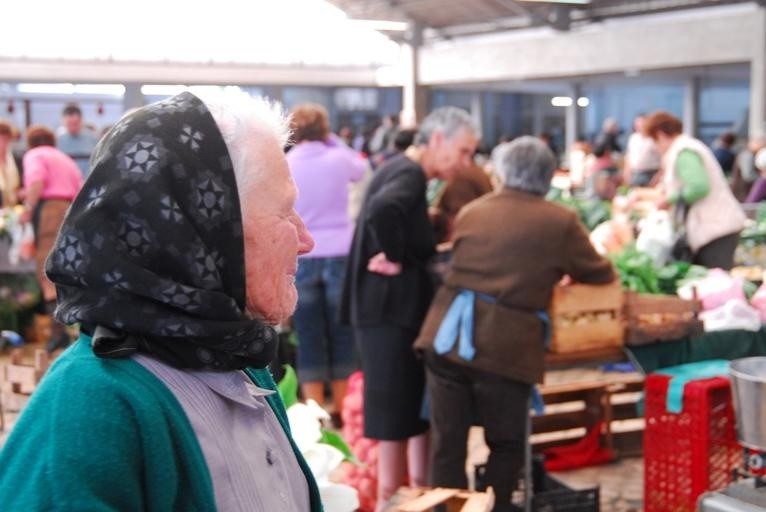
[338,370,380,511]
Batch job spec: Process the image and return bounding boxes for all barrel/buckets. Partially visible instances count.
[732,355,761,451]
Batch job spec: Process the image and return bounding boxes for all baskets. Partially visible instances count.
[642,371,735,512]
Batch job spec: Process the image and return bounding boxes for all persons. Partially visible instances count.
[0,83,326,511]
[410,134,620,512]
[268,102,765,425]
[334,106,485,511]
[1,104,113,355]
